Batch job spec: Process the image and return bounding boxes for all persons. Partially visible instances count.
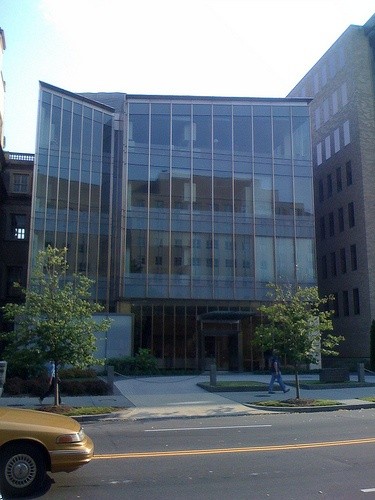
[269,349,290,394]
[38,359,64,405]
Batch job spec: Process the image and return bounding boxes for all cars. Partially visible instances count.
[0,403,94,498]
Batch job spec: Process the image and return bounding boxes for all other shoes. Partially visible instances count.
[39,399,43,404]
[284,389,290,393]
[269,391,275,394]
[55,402,65,405]
